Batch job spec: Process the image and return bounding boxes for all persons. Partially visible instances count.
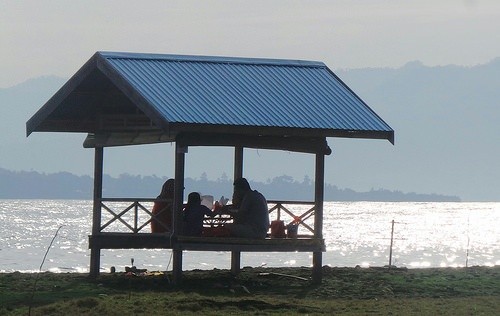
[151,179,175,233]
[222,177,269,239]
[182,192,219,237]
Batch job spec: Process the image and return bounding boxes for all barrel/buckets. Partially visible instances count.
[287,225,298,238]
